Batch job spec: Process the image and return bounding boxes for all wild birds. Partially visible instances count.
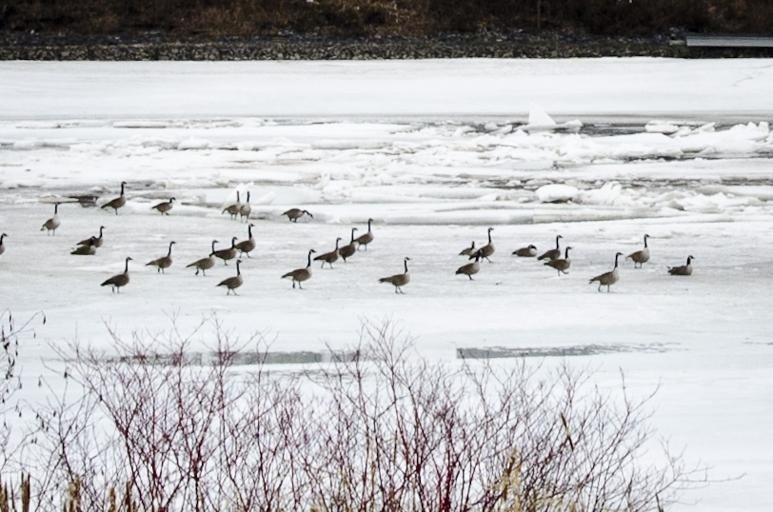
[0,231,7,257]
[38,203,63,235]
[378,258,413,292]
[144,240,177,275]
[70,225,105,256]
[278,207,377,289]
[151,197,177,218]
[100,257,131,294]
[185,223,258,297]
[100,180,127,215]
[222,191,253,222]
[455,221,697,295]
